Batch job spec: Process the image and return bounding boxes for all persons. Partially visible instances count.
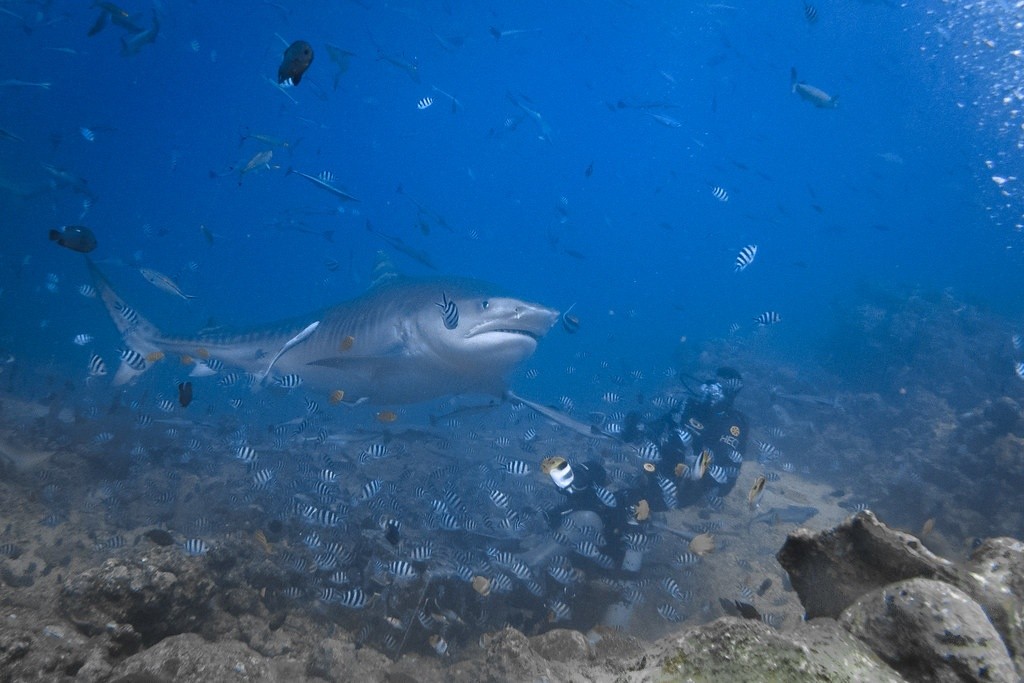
[487,366,750,585]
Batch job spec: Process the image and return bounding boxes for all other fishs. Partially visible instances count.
[0,0,1023,665]
